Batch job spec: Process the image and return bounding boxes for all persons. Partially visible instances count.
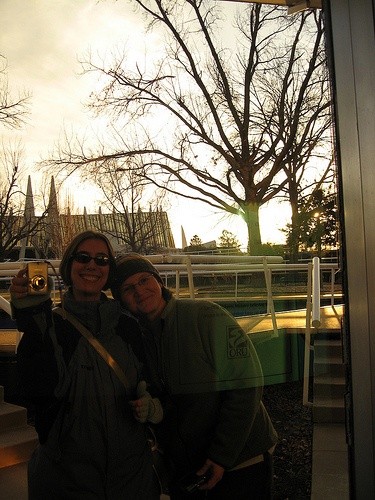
[108,254,278,500]
[10,230,166,500]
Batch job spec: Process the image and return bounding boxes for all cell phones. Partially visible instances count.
[189,465,210,496]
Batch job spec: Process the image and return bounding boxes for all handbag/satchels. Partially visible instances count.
[146,426,157,455]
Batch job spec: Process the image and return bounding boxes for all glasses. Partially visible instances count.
[119,273,154,298]
[73,253,111,266]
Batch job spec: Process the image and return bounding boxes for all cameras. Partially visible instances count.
[25,260,48,294]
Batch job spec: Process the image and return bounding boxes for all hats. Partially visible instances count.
[110,251,164,302]
